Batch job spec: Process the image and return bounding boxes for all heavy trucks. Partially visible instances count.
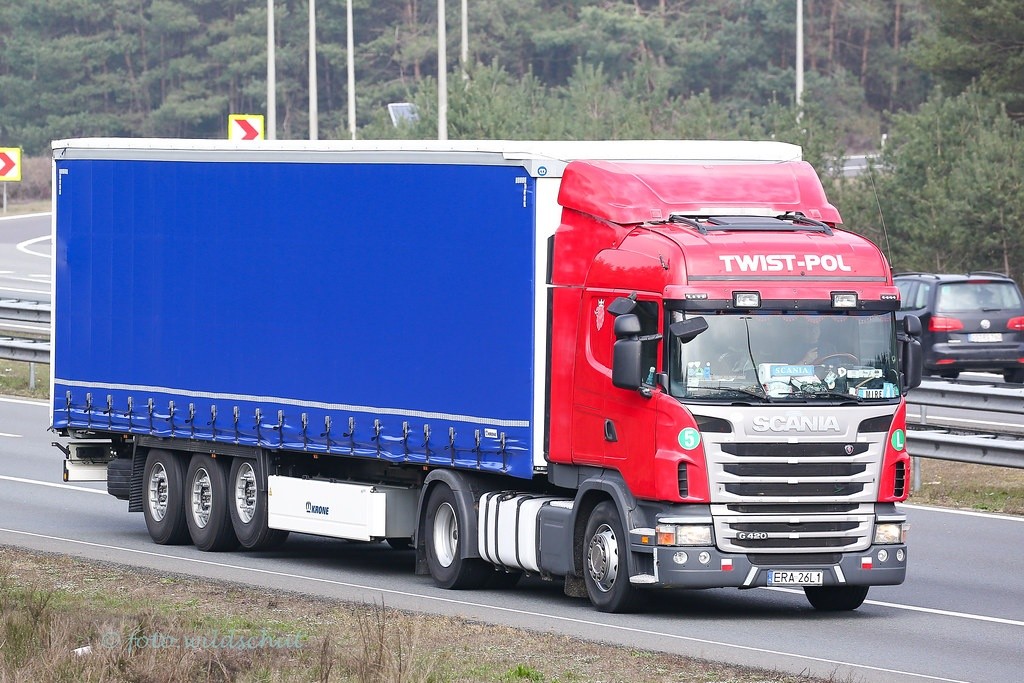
[47,138,922,615]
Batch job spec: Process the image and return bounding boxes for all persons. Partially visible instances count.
[785,318,840,365]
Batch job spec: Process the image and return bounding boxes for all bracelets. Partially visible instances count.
[801,359,809,365]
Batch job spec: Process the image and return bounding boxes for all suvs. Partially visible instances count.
[892,271,1024,383]
